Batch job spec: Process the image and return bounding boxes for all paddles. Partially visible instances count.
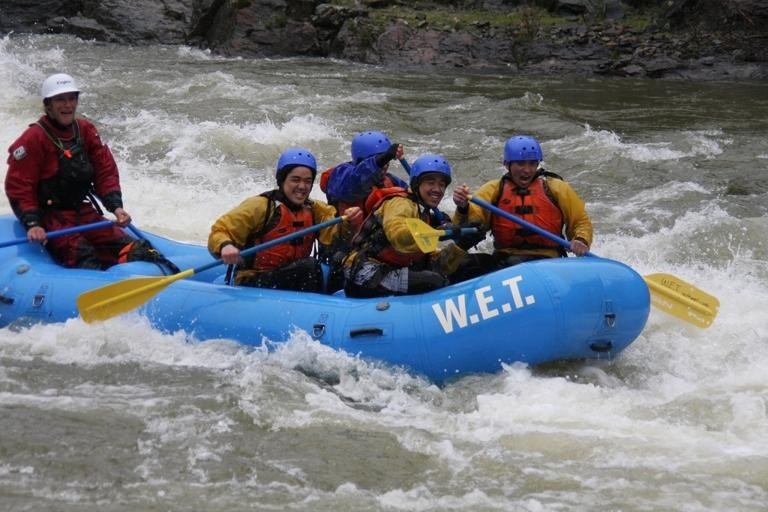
[77,213,352,327]
[404,218,478,255]
[462,184,721,328]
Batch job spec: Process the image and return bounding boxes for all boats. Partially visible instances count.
[0,207,649,376]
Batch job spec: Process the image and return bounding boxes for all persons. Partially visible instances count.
[339,153,498,298]
[452,133,593,267]
[206,148,364,291]
[4,73,145,272]
[321,129,452,261]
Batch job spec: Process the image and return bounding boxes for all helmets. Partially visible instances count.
[410,155,451,185]
[41,73,80,102]
[351,132,392,166]
[276,149,316,178]
[503,135,542,166]
